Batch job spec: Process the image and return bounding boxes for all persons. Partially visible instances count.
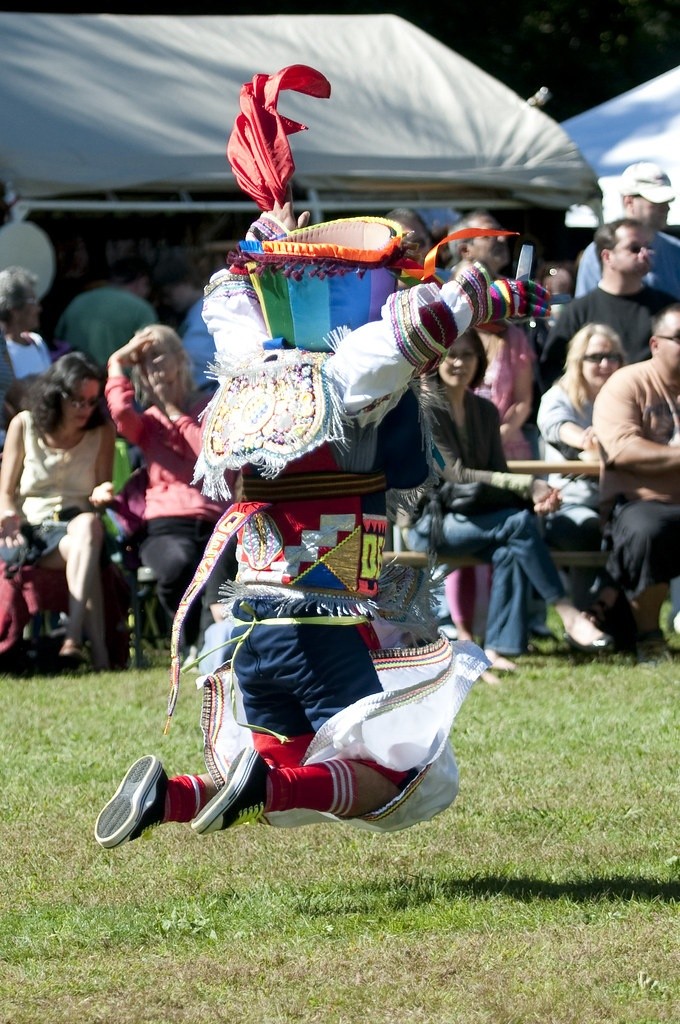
[0,162,680,670]
[93,201,550,850]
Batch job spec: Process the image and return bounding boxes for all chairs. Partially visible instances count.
[1,556,131,669]
[106,464,156,669]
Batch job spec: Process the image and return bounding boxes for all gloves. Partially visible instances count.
[490,277,552,319]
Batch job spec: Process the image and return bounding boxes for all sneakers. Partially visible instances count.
[191,747,271,836]
[95,756,171,849]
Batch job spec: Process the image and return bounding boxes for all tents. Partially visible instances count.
[0,11,605,227]
[557,64,680,227]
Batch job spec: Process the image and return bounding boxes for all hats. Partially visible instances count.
[619,161,676,203]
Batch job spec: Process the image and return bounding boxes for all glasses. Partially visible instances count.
[615,243,654,254]
[68,396,100,409]
[584,352,624,363]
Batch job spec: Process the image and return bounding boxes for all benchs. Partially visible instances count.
[382,460,611,647]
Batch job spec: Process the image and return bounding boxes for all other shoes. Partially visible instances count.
[563,628,618,653]
[637,627,665,645]
